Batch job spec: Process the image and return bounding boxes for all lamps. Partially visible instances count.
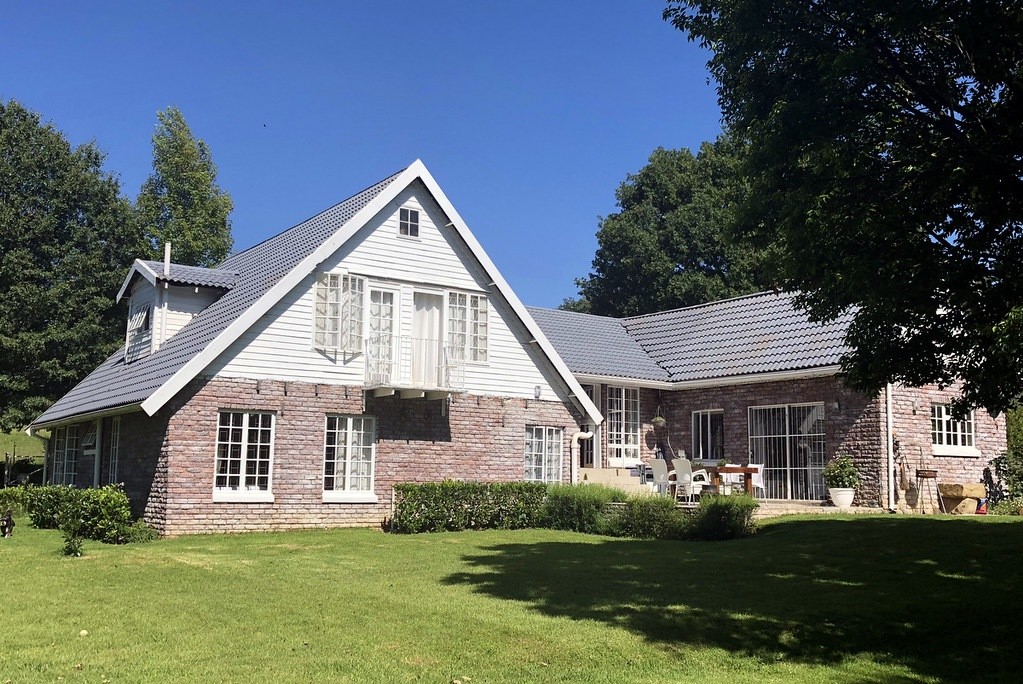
[832,399,840,412]
[535,385,542,398]
[913,397,919,410]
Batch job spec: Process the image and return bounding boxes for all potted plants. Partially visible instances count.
[652,417,667,429]
[718,459,730,467]
[821,456,862,506]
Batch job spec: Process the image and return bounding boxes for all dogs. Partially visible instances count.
[0,513,15,539]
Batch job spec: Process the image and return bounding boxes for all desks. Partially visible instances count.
[666,465,759,505]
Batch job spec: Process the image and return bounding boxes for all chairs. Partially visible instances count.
[646,459,768,506]
